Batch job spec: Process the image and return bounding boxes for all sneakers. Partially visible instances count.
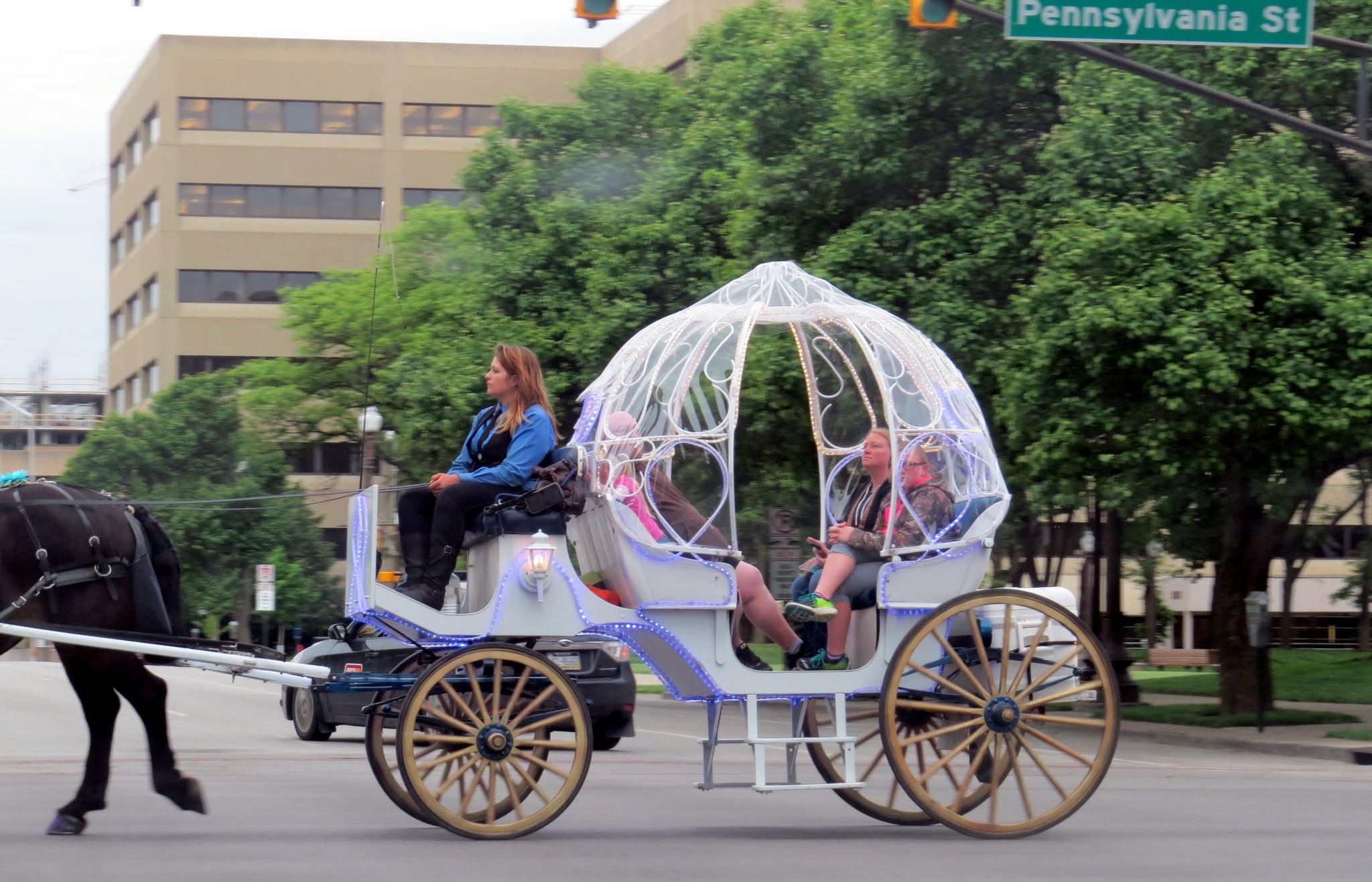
[796,650,852,670]
[784,640,819,671]
[734,641,773,671]
[784,592,837,623]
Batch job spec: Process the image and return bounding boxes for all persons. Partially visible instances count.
[782,438,963,670]
[390,342,565,611]
[600,412,822,672]
[782,424,901,670]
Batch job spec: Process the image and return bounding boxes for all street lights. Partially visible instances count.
[1075,528,1097,629]
[358,405,384,491]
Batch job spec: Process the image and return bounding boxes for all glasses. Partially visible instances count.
[888,461,927,470]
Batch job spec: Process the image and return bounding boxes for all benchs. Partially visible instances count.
[564,488,741,675]
[829,491,1010,672]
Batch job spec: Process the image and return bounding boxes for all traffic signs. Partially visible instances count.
[1005,0,1314,49]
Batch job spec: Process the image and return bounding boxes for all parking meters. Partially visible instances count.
[1244,591,1269,733]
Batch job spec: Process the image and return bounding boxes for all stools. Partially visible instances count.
[459,445,583,615]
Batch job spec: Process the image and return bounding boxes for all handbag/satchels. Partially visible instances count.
[483,482,565,515]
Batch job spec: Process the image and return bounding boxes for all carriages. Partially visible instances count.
[0,258,1124,841]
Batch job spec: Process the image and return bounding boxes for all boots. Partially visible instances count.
[400,534,464,611]
[393,528,433,593]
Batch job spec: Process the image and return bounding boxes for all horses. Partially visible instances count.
[0,483,207,835]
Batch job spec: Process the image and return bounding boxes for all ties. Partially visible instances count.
[477,408,503,452]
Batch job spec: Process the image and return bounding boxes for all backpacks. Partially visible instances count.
[531,458,586,516]
